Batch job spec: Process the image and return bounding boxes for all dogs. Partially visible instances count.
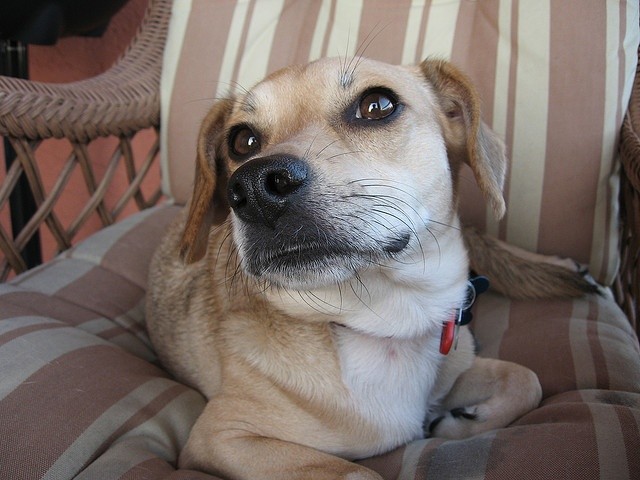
[142,19,612,480]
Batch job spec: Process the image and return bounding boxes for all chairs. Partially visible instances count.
[0,0,639,479]
